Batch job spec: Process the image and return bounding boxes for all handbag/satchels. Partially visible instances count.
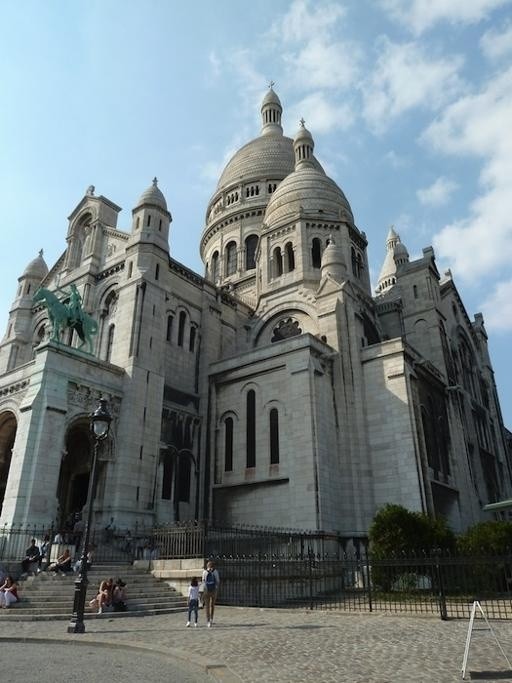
[89,599,97,608]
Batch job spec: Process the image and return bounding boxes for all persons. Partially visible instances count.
[112,577,131,611]
[57,282,83,326]
[203,558,222,628]
[1,575,21,608]
[18,514,94,575]
[185,577,203,628]
[122,529,164,559]
[89,576,114,615]
[104,515,116,543]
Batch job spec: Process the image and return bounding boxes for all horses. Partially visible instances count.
[32,287,98,355]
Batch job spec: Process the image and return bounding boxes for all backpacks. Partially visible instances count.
[206,568,216,592]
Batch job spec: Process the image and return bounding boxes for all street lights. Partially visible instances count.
[68,397,113,633]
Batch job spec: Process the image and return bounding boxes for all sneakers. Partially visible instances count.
[186,622,198,627]
[207,620,213,628]
